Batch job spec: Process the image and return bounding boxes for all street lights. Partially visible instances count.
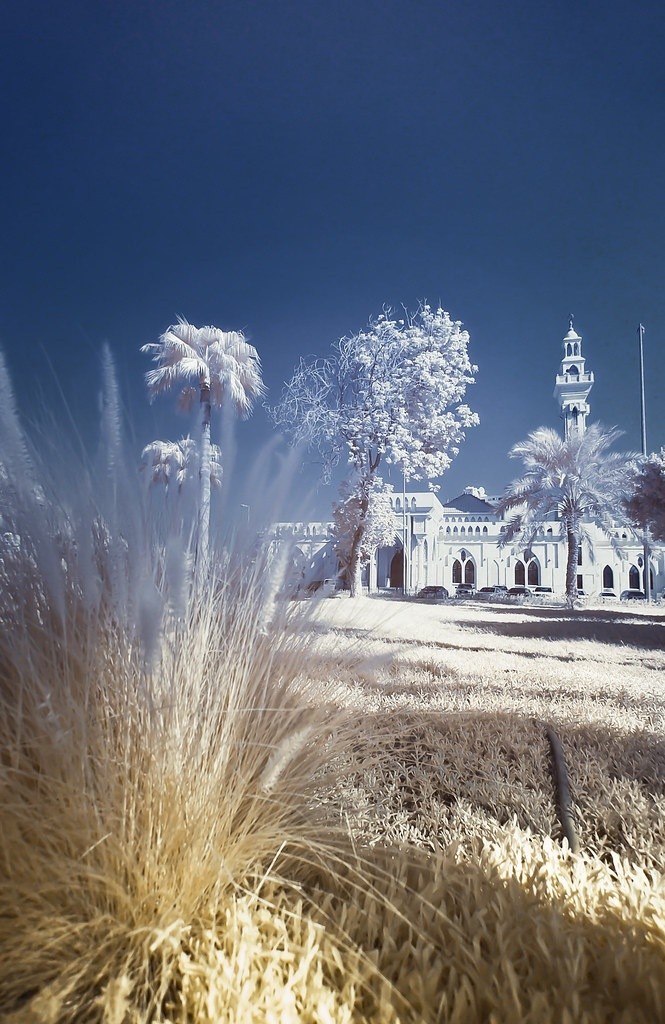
[636,322,651,603]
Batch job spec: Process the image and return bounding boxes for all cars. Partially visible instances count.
[477,586,502,600]
[418,586,449,599]
[493,585,509,594]
[619,589,645,601]
[506,587,530,596]
[600,591,616,599]
[577,589,589,599]
[532,586,553,596]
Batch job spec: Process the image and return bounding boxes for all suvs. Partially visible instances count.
[455,583,476,599]
